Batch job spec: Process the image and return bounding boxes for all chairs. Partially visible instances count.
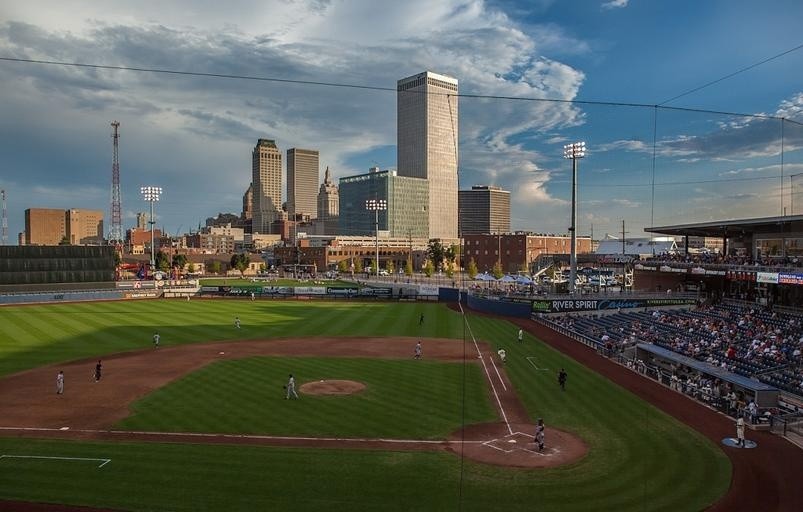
[550,303,803,430]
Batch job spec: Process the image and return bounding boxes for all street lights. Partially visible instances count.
[563,140,586,293]
[365,193,387,278]
[140,185,163,270]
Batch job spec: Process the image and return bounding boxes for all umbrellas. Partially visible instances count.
[472,273,535,291]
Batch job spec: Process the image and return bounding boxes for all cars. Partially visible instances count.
[543,275,617,293]
[378,270,390,276]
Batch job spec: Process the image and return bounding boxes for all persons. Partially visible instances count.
[153,332,160,352]
[252,292,255,301]
[735,413,746,449]
[56,370,64,394]
[415,340,422,360]
[518,327,523,344]
[419,312,424,326]
[93,359,103,383]
[498,347,506,365]
[233,317,241,329]
[540,251,802,425]
[535,418,545,453]
[473,283,534,296]
[285,374,298,400]
